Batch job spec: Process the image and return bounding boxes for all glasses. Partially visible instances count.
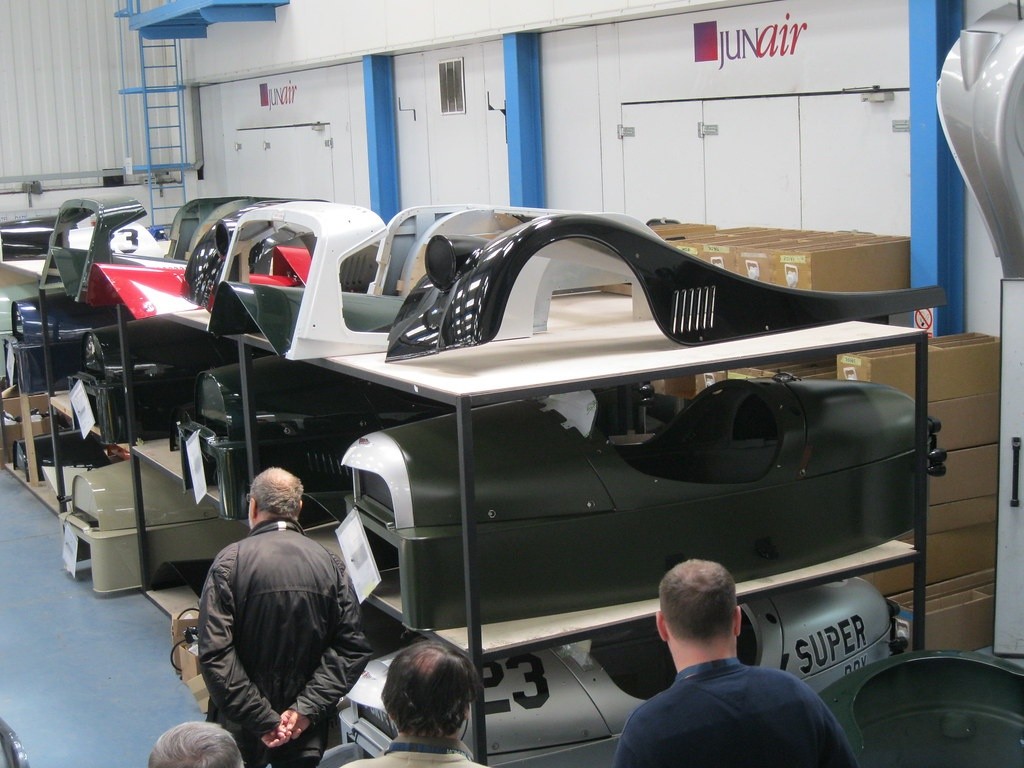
[247,494,252,502]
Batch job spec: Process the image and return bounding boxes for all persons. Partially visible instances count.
[197,466,363,768]
[338,640,489,768]
[148,720,245,768]
[611,559,859,768]
